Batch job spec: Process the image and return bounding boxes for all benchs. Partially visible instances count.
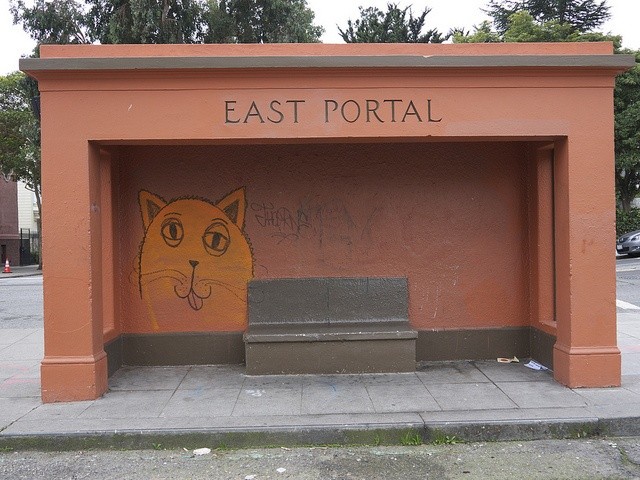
[242,277,419,376]
